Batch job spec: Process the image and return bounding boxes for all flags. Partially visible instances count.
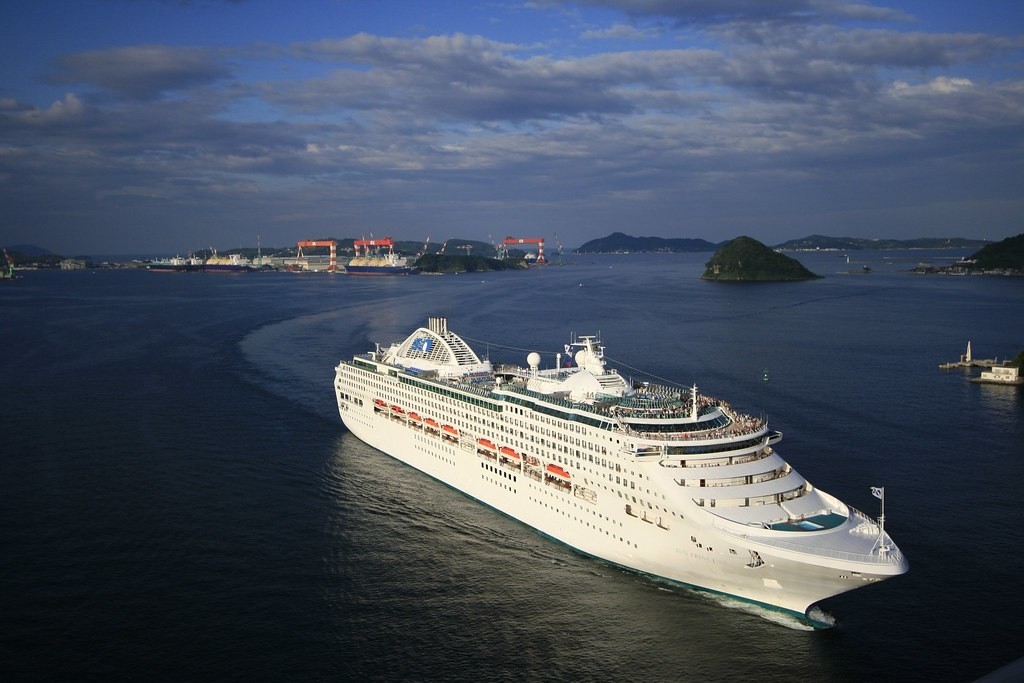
[564,345,573,357]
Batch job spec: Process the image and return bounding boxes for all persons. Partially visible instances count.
[602,392,761,443]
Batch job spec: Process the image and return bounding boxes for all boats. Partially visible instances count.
[144,232,415,274]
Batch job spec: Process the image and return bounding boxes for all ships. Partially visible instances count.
[335,316,912,634]
